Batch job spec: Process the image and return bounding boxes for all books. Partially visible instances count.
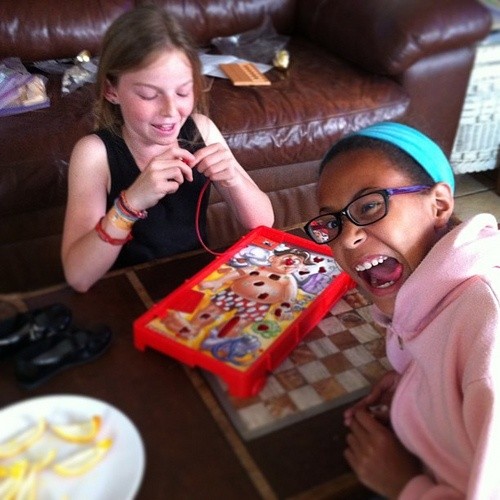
[219,61,272,87]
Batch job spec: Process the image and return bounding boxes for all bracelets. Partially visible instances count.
[96,190,148,246]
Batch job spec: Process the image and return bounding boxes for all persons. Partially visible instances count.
[316,117,500,500]
[60,0,276,294]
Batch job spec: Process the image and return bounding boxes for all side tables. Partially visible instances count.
[449,9,500,196]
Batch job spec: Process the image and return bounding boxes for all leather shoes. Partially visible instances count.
[1,303,72,359]
[13,325,113,387]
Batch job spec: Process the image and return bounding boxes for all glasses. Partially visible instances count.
[304,185,430,245]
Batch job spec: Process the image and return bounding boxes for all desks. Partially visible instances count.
[1,224,366,500]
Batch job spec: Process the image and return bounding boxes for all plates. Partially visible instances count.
[0,394,145,500]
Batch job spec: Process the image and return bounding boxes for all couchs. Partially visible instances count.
[0,0,493,294]
